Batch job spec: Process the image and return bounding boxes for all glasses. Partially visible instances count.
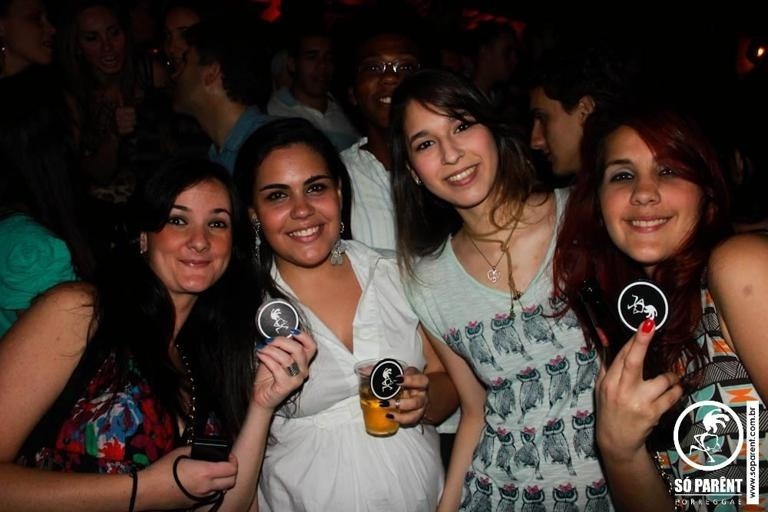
[357,59,419,78]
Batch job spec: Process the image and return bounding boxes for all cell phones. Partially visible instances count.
[191,438,230,461]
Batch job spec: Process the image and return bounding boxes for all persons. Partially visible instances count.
[3,2,768,511]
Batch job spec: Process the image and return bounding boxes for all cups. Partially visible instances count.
[355,354,409,438]
